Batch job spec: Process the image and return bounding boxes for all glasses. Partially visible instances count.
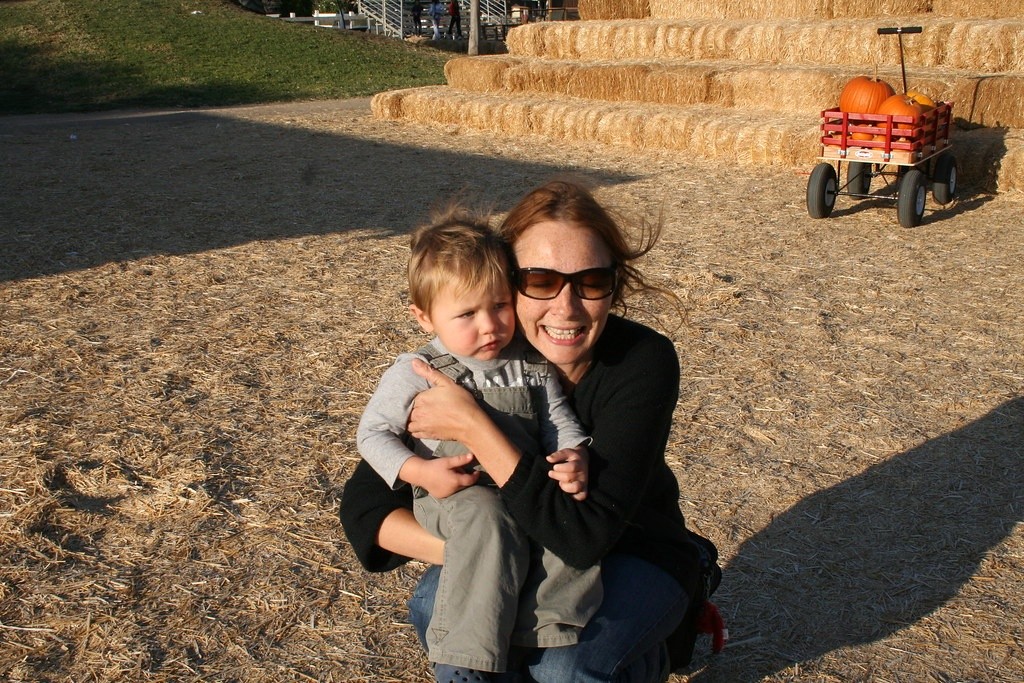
[512,267,619,300]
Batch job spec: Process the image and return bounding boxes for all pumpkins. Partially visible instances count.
[830,64,938,152]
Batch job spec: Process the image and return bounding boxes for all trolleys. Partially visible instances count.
[806,27,958,229]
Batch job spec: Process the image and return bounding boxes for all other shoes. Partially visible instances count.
[699,600,725,652]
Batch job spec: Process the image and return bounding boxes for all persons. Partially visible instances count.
[339,180,723,683]
[356,209,605,682]
[427,0,444,40]
[411,0,425,37]
[446,0,462,39]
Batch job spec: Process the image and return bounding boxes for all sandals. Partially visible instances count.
[428,658,483,683]
